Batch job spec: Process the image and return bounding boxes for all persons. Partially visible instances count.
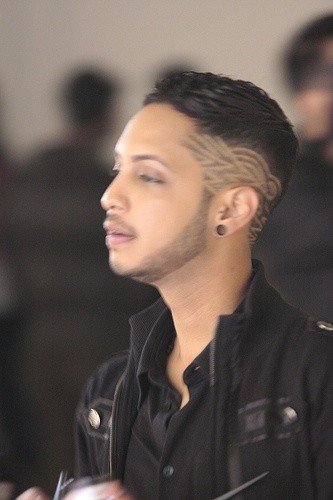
[1,11,333,500]
